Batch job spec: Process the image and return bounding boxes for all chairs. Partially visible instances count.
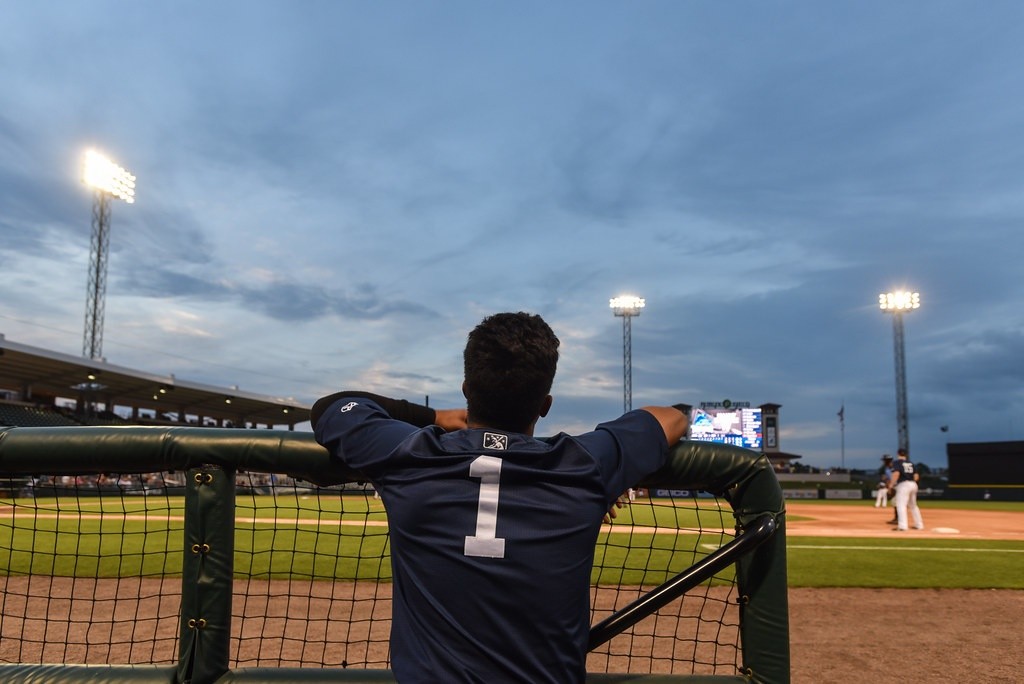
[0,402,128,427]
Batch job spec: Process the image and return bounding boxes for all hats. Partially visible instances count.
[881,454,892,460]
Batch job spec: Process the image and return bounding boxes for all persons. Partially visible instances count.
[310,313,690,684]
[874,448,924,531]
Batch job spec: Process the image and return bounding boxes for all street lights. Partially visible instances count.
[877,290,922,462]
[609,293,647,416]
[80,145,138,411]
